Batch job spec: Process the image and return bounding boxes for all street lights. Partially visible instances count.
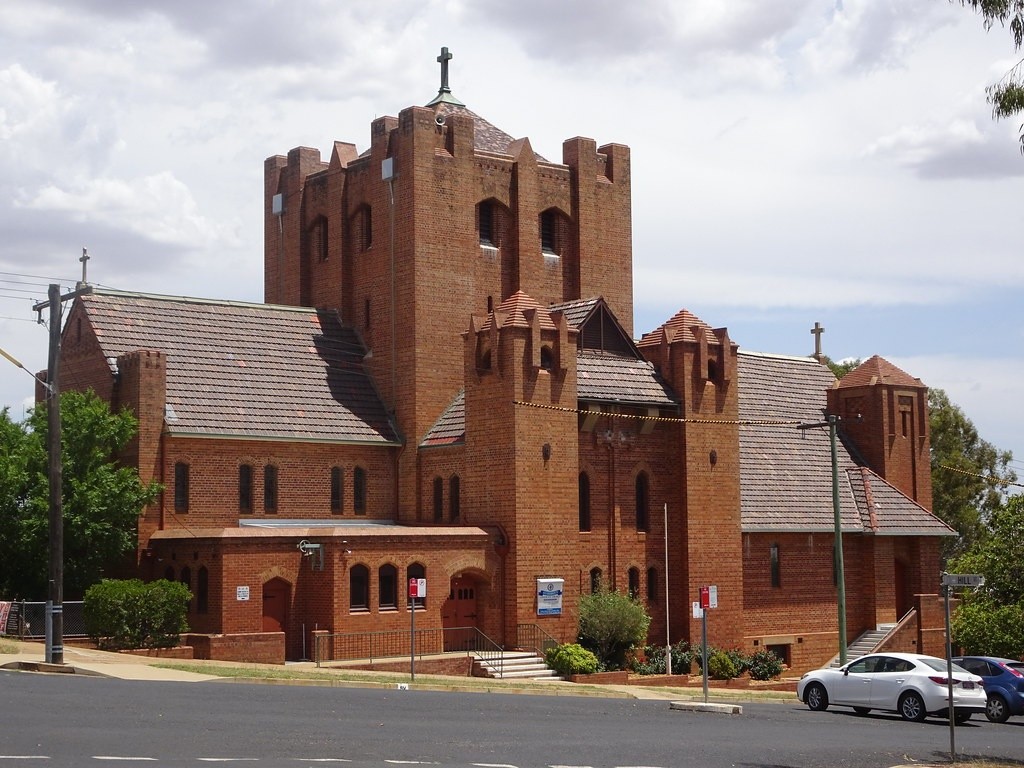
[1,348,73,673]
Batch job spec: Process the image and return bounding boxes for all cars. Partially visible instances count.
[942,655,1024,723]
[796,652,988,726]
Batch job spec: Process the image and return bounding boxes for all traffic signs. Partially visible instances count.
[940,573,986,588]
